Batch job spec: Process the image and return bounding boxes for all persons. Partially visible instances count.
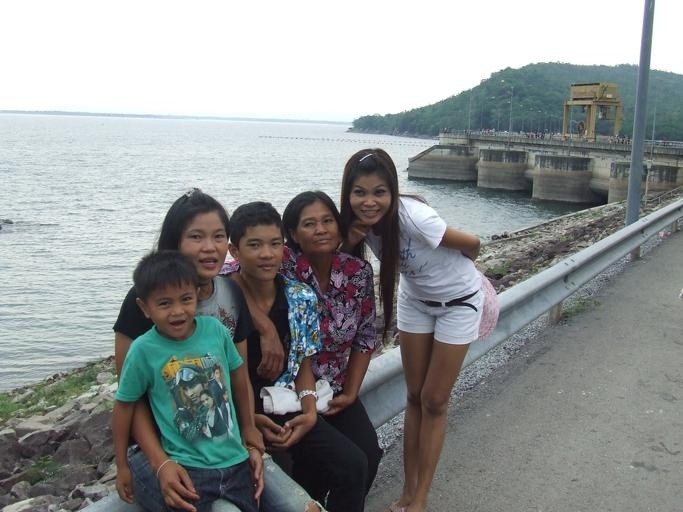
[440,127,670,144]
[228,202,351,511]
[220,191,380,511]
[114,190,324,512]
[116,251,266,512]
[340,150,483,511]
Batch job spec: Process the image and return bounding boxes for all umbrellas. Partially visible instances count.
[386,501,409,512]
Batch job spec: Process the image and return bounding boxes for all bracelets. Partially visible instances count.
[299,390,319,400]
[156,459,178,479]
[247,446,255,451]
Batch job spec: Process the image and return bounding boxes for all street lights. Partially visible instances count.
[475,270,501,338]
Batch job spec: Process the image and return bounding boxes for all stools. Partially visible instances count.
[418,289,479,312]
